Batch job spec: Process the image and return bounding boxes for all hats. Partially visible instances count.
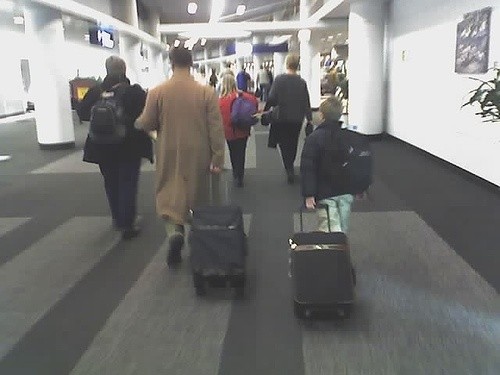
[106,56,126,75]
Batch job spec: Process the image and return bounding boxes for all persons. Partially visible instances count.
[208,66,218,88]
[215,73,264,189]
[300,95,366,287]
[133,44,226,272]
[265,65,274,97]
[321,62,348,100]
[257,52,314,187]
[74,54,157,239]
[236,64,252,93]
[256,64,271,101]
[219,60,235,79]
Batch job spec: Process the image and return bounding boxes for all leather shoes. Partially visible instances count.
[123,227,142,238]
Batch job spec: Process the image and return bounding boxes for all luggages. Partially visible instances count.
[188,169,248,296]
[289,202,357,321]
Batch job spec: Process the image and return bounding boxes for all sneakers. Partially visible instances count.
[168,236,185,268]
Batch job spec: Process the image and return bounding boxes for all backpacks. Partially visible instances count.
[329,143,373,195]
[230,92,259,126]
[89,83,127,145]
